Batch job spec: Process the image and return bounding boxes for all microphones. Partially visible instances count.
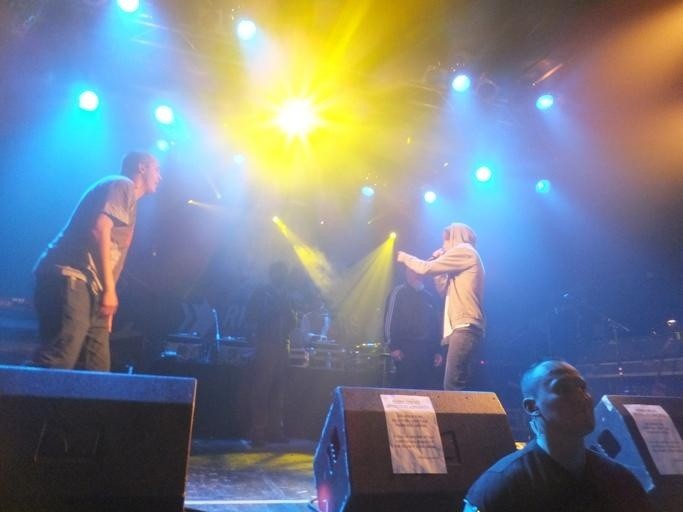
[426,247,445,263]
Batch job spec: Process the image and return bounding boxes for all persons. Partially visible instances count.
[462,356,658,511]
[240,261,299,446]
[383,266,446,388]
[394,221,488,390]
[30,153,163,372]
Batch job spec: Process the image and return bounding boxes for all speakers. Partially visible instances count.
[0,362,199,512]
[311,384,520,511]
[583,394,683,500]
